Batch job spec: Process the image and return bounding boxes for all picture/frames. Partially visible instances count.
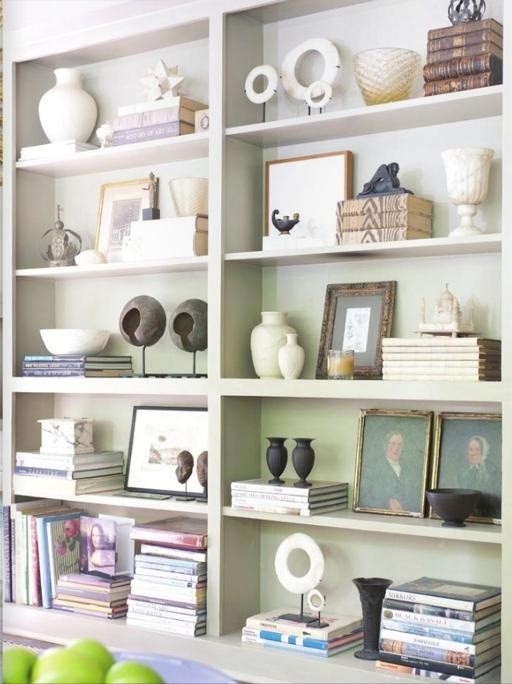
[352,407,432,519]
[428,410,503,524]
[93,178,157,264]
[123,406,207,500]
[315,281,396,380]
[263,151,351,237]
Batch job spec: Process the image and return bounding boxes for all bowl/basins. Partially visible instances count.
[427,489,482,526]
[38,327,111,357]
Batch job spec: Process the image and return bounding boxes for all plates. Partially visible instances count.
[112,650,241,684]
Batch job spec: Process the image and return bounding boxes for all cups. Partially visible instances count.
[353,46,422,105]
[167,175,210,218]
[325,349,355,381]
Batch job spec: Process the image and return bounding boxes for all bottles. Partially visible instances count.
[292,437,316,487]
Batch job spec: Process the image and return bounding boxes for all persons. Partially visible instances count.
[449,436,498,496]
[364,429,419,510]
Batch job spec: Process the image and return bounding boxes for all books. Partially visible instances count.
[21,352,135,379]
[2,499,209,637]
[121,233,209,259]
[11,449,128,498]
[377,575,502,682]
[335,225,434,246]
[17,136,102,164]
[108,96,209,148]
[128,214,208,235]
[240,607,363,657]
[335,209,434,233]
[423,18,503,96]
[380,337,501,383]
[231,475,350,517]
[335,191,433,217]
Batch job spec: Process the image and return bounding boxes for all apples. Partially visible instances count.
[71,640,117,673]
[2,645,37,684]
[103,660,163,684]
[29,646,103,684]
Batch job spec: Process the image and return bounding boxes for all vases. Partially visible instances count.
[288,438,315,489]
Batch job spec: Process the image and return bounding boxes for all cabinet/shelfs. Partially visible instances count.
[0,0,512,684]
